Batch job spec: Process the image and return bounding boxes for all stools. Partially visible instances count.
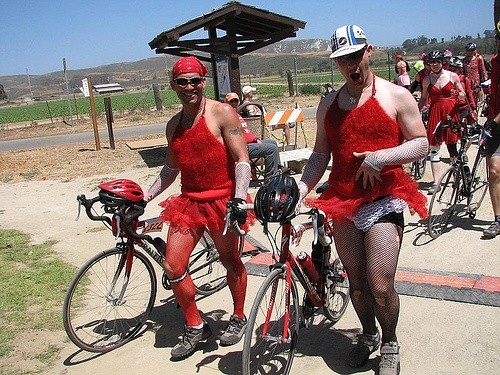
[248,157,267,189]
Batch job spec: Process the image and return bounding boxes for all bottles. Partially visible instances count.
[464,166,472,181]
[153,236,167,255]
[297,251,320,281]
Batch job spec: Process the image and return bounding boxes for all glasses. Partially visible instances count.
[173,76,203,87]
[228,98,239,103]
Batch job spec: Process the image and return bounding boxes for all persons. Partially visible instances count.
[225,93,280,185]
[295,23,428,375]
[143,56,252,360]
[394,55,412,91]
[413,42,490,195]
[479,32,500,238]
[242,85,291,145]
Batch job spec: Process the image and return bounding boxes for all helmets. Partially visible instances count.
[98,178,144,204]
[417,43,477,67]
[253,174,299,222]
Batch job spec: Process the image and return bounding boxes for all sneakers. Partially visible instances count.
[171,319,212,358]
[220,314,248,344]
[378,340,400,375]
[345,327,380,368]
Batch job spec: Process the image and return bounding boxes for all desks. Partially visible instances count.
[264,109,309,151]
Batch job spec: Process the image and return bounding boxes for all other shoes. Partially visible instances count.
[278,164,290,174]
[483,222,500,238]
[450,156,459,168]
[430,152,440,161]
[428,183,441,194]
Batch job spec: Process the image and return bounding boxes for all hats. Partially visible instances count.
[226,92,240,102]
[242,85,257,95]
[330,24,368,58]
[396,55,402,58]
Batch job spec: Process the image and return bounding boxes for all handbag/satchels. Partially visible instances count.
[461,92,478,125]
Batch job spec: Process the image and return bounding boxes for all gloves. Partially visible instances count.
[229,198,248,225]
[134,199,148,209]
